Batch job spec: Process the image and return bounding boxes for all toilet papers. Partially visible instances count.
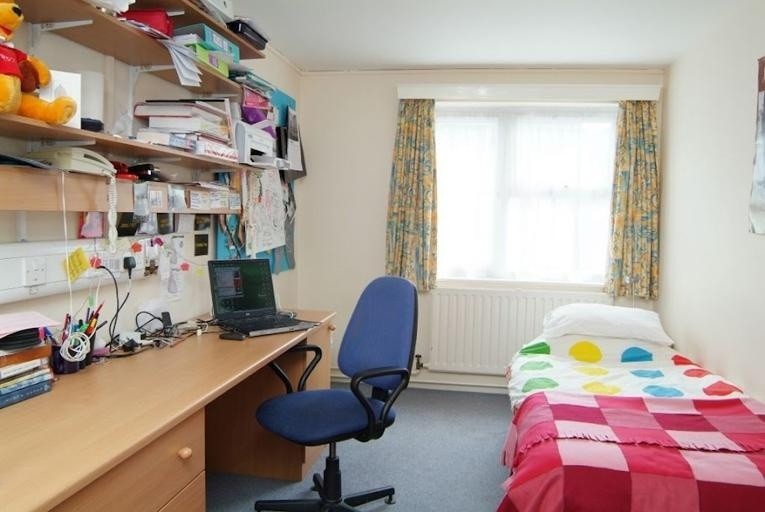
[78,71,105,122]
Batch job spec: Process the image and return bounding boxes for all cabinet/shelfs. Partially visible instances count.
[0,309,338,512]
[0,0,266,170]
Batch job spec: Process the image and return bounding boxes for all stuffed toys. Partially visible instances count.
[0,0,77,127]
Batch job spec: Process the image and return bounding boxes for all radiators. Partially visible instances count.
[424,287,613,377]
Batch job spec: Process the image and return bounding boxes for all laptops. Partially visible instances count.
[207,259,314,337]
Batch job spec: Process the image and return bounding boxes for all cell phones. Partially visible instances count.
[218,332,246,340]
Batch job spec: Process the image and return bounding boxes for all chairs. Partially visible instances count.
[253,277,418,512]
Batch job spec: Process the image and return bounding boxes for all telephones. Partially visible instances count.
[26,146,117,177]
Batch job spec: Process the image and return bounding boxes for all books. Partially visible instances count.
[133,99,239,164]
[0,311,59,409]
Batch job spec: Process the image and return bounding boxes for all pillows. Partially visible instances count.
[539,302,674,347]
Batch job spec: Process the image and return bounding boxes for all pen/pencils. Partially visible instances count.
[44,327,58,345]
[62,303,107,345]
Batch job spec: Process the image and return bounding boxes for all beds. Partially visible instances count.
[507,339,765,512]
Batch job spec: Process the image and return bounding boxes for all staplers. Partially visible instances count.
[128,164,160,182]
